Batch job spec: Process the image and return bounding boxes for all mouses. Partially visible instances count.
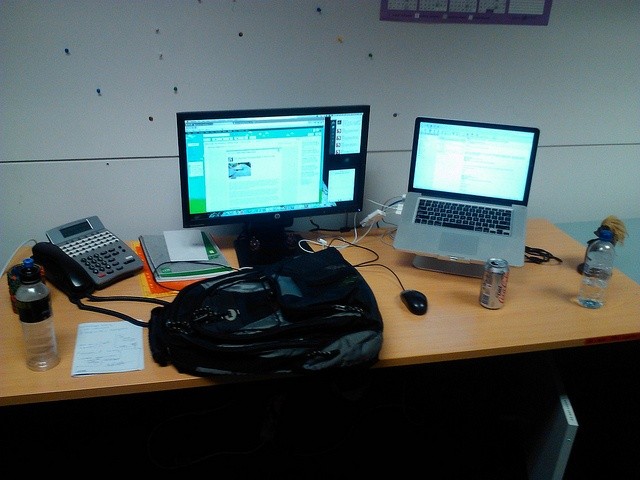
[400,289,428,316]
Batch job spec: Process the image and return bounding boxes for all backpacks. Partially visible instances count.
[149,247,385,395]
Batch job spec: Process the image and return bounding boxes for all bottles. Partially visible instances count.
[16,259,60,371]
[580,229,614,307]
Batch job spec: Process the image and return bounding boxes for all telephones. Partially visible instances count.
[30,216,144,298]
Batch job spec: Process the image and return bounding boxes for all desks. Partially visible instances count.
[0,219,639,406]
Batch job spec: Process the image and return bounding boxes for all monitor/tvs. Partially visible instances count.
[176,104,371,268]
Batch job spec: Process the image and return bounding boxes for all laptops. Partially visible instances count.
[391,116,540,269]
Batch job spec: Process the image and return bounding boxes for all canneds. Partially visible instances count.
[479,258,509,309]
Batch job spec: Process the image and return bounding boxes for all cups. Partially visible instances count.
[7,263,45,314]
